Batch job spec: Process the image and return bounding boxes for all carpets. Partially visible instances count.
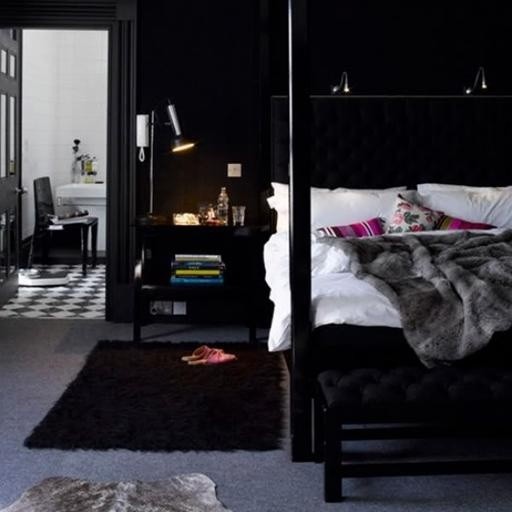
[0,472,232,512]
[22,338,287,455]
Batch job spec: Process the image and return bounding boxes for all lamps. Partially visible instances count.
[331,72,352,95]
[461,66,488,94]
[136,96,197,226]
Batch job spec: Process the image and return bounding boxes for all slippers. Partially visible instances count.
[181,345,238,366]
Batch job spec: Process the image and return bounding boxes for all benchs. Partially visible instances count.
[312,364,512,502]
[134,276,259,343]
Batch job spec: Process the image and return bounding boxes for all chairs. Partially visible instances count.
[28,176,98,276]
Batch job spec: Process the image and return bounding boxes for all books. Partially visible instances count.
[171,254,225,284]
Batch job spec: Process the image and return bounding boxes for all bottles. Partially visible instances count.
[216,187,229,226]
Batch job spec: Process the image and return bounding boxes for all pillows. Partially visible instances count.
[266,177,512,238]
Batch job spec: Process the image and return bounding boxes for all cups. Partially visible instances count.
[232,206,245,227]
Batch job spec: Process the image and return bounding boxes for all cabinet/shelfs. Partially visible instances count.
[129,224,275,328]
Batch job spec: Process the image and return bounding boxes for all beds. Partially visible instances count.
[269,94,512,463]
